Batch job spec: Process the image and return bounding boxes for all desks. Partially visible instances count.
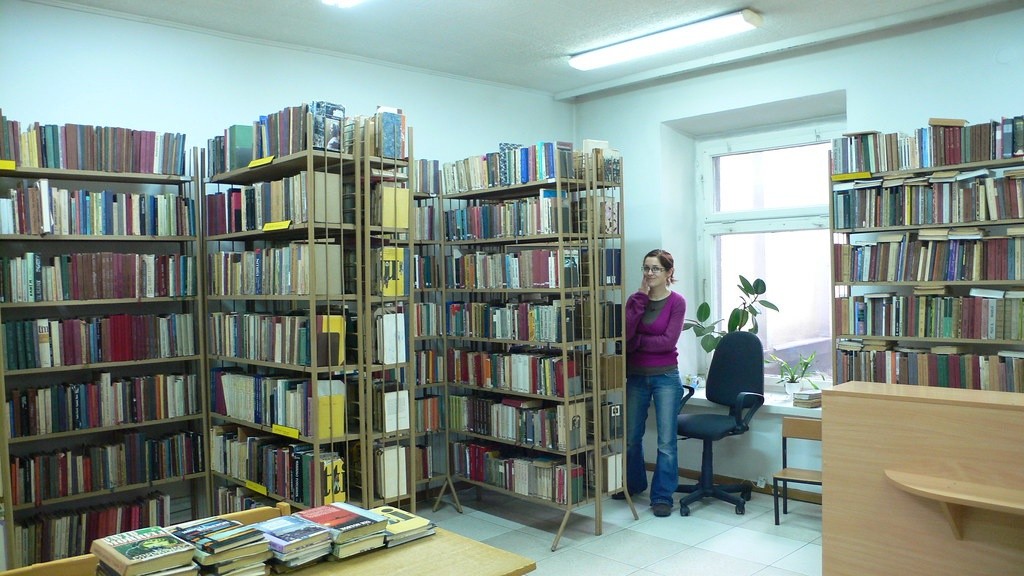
[821,380,1024,576]
[286,528,536,576]
[683,387,822,418]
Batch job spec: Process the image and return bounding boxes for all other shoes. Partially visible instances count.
[612,487,644,500]
[653,503,670,517]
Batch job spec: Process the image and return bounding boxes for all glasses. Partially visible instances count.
[641,267,666,272]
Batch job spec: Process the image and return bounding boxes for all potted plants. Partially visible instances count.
[765,351,825,396]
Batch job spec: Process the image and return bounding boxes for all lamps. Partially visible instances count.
[568,9,763,71]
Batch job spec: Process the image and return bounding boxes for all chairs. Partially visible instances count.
[773,417,822,525]
[220,502,291,528]
[0,553,99,576]
[676,331,764,517]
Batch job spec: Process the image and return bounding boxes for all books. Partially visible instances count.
[90,502,438,576]
[831,116,1024,392]
[209,99,407,516]
[413,139,622,504]
[1,107,207,569]
[793,390,821,408]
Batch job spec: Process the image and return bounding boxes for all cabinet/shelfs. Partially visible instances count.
[0,98,639,550]
[827,115,1024,393]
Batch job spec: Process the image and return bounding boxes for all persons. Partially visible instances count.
[611,249,686,516]
